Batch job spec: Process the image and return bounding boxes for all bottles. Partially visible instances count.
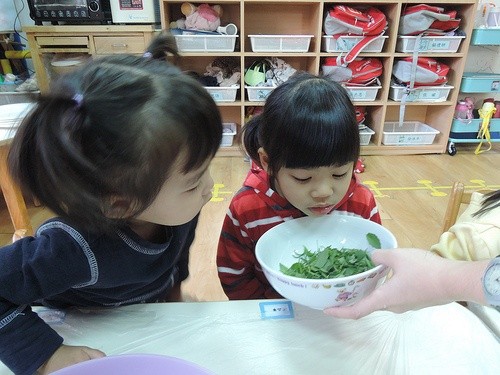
[453,100,467,120]
[492,100,500,118]
[463,98,474,119]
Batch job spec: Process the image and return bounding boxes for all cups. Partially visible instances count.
[473,0,500,29]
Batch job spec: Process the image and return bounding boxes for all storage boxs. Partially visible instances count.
[173,31,239,54]
[245,84,277,102]
[359,124,375,145]
[389,83,455,103]
[449,112,500,143]
[460,72,500,93]
[203,82,240,103]
[396,31,466,53]
[470,28,500,46]
[382,121,441,146]
[345,80,383,102]
[246,31,315,52]
[221,122,238,147]
[322,32,390,53]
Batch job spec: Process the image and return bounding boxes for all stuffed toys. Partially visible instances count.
[169,3,224,32]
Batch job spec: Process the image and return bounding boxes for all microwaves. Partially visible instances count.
[27,0,110,24]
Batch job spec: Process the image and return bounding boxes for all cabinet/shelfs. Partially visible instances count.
[160,0,479,157]
[21,25,154,98]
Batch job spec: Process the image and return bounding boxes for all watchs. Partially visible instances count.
[480,257,500,307]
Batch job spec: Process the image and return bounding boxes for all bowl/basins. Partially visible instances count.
[255,214,397,311]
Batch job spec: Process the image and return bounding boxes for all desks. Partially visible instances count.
[0,102,42,239]
[0,270,500,375]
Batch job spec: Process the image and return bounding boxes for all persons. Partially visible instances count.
[323,247,500,320]
[215,71,383,301]
[0,30,222,375]
[428,191,500,261]
